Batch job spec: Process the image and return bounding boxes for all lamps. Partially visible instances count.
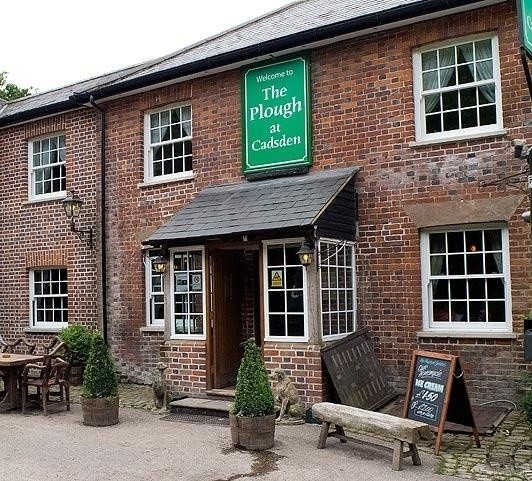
[62,186,94,249]
[294,229,323,272]
[152,245,170,272]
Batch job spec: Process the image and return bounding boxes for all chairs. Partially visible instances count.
[0,333,74,417]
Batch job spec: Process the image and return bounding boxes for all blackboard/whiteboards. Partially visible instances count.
[403,350,456,432]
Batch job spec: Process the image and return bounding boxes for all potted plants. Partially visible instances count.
[228,338,278,451]
[59,322,120,426]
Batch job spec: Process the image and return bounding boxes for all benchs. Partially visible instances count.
[308,400,429,471]
